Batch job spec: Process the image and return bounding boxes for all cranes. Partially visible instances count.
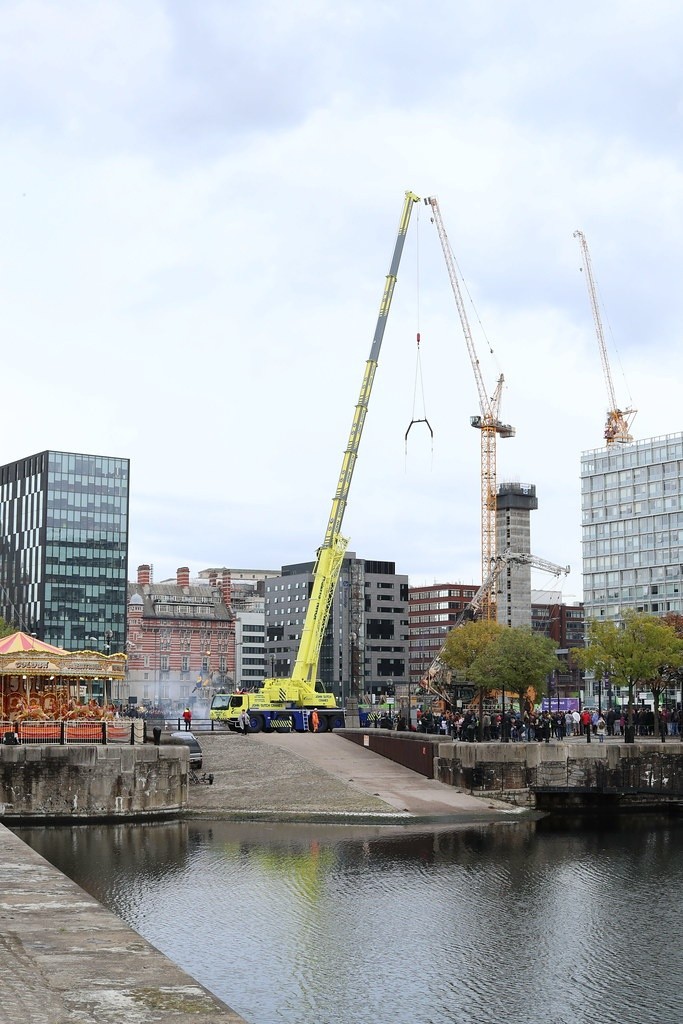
[423,192,507,628]
[572,225,637,448]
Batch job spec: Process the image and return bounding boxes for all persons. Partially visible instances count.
[111,703,249,735]
[395,704,683,742]
[312,709,319,732]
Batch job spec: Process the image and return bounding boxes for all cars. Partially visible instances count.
[183,737,204,769]
[169,730,194,740]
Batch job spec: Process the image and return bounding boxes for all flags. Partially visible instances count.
[192,673,255,697]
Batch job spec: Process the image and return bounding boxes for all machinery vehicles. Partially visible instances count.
[422,549,571,720]
[207,191,419,733]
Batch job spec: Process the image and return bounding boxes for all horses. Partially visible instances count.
[15,698,115,724]
